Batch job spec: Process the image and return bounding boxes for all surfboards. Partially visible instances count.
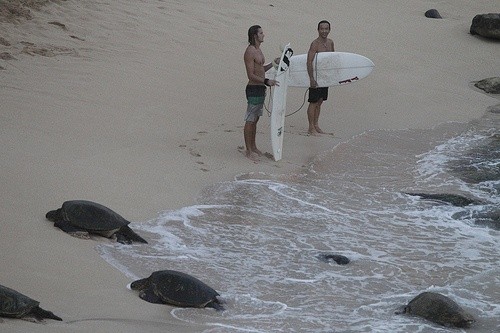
[269,41,295,161]
[264,52,375,88]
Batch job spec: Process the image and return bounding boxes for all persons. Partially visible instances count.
[244,24,281,161]
[307,20,334,136]
